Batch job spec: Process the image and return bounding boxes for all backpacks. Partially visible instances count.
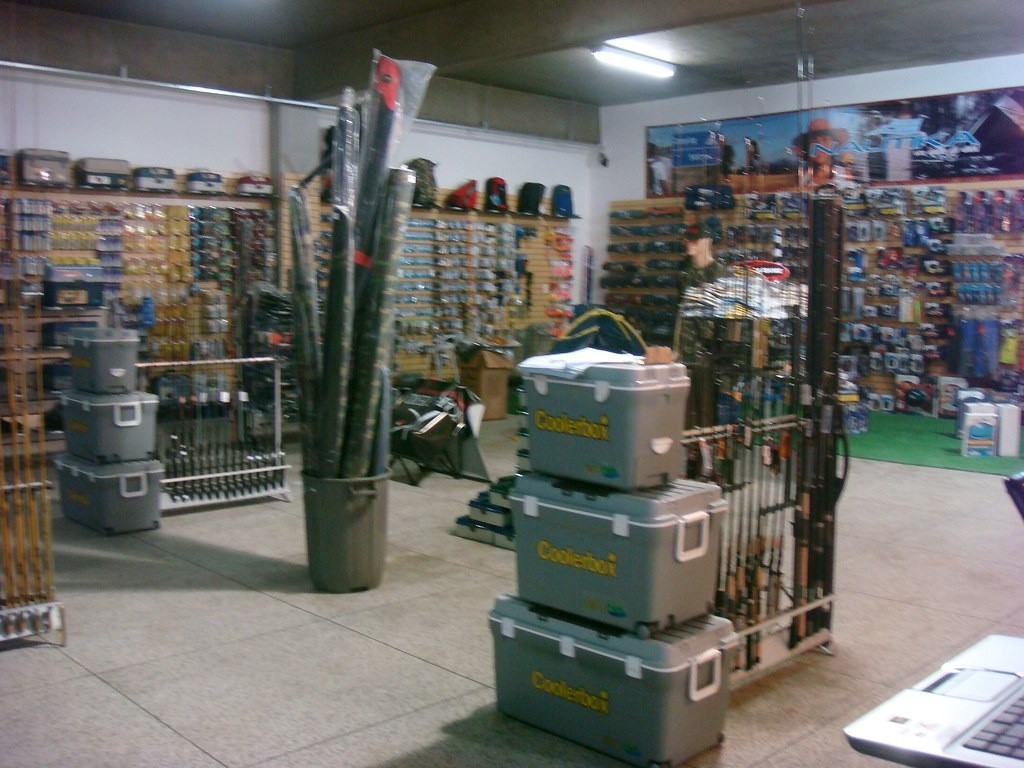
[485,177,509,210]
[519,183,545,213]
[553,185,572,215]
[405,158,437,204]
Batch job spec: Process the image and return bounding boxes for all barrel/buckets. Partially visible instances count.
[298,467,393,594]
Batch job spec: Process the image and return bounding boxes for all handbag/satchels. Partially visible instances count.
[448,180,476,206]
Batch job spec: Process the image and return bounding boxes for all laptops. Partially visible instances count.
[841,473,1024,767]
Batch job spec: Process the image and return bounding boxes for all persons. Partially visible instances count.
[677,224,723,290]
[791,118,852,184]
[744,136,760,173]
[645,142,669,197]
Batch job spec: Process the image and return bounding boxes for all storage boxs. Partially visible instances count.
[508,474,723,637]
[42,266,105,307]
[0,154,11,186]
[60,390,161,465]
[185,173,226,195]
[454,349,515,423]
[55,451,165,540]
[77,158,131,192]
[518,360,693,492]
[236,175,274,195]
[17,150,71,188]
[836,186,1024,457]
[133,168,175,193]
[69,327,141,395]
[41,323,98,348]
[487,589,740,768]
[43,364,94,391]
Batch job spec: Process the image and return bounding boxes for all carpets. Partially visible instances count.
[837,412,1024,476]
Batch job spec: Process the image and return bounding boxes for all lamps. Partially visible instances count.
[587,44,677,81]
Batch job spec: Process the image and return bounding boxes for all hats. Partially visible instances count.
[793,118,850,156]
[683,223,714,240]
